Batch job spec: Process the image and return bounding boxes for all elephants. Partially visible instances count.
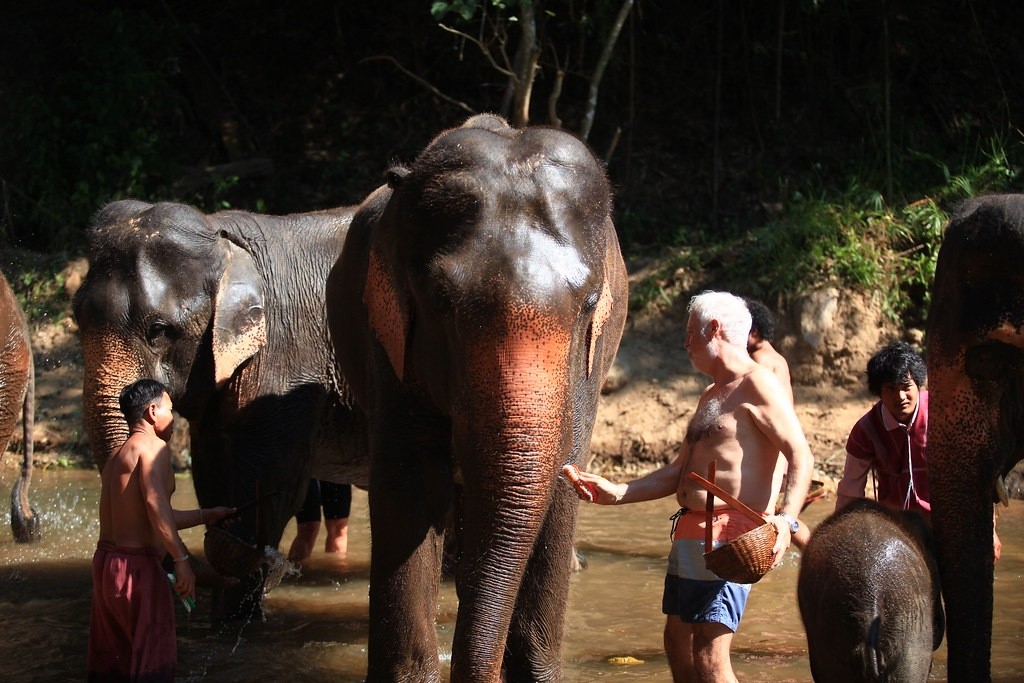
[795,193,1024,683]
[0,112,631,682]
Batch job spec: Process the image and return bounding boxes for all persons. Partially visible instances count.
[86,378,241,683]
[744,297,793,405]
[575,288,814,683]
[287,479,352,561]
[834,341,1002,564]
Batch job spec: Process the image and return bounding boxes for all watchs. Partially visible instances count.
[777,512,799,534]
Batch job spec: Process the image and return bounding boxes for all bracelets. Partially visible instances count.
[173,553,192,563]
[198,509,203,525]
